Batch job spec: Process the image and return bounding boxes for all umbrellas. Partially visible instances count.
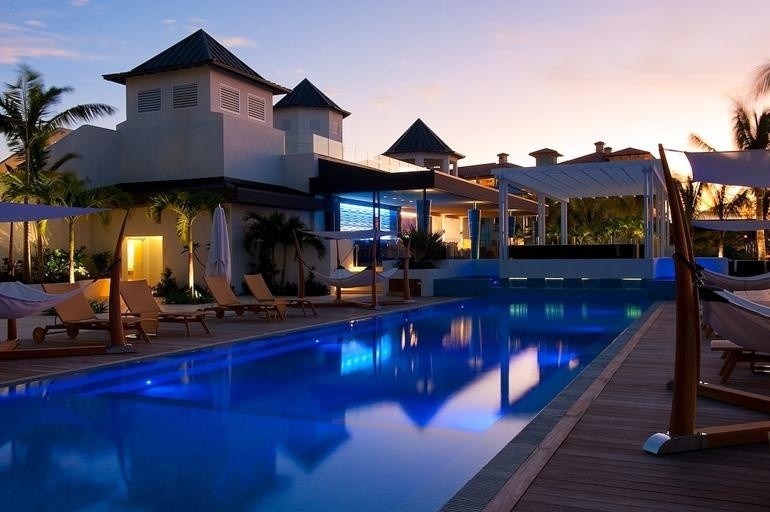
[206,201,232,288]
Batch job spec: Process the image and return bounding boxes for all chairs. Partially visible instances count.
[38,273,318,341]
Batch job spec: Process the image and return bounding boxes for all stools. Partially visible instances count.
[710,338,769,388]
